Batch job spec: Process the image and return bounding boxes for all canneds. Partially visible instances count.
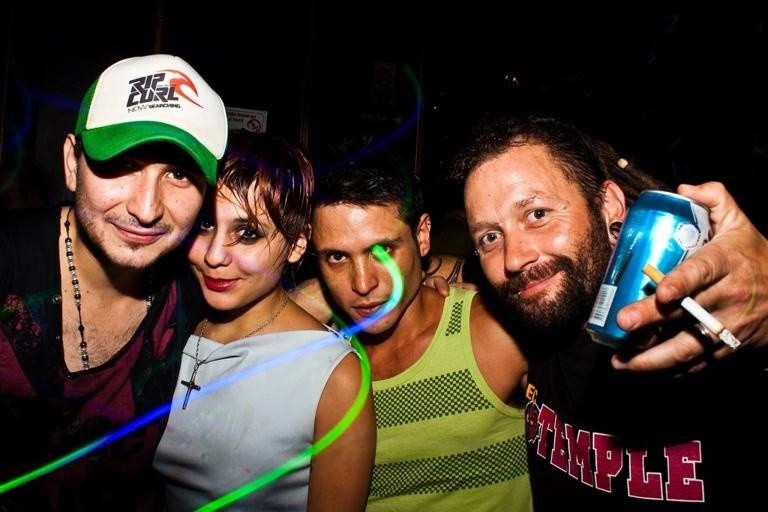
[584,190,714,362]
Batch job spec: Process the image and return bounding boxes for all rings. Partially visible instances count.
[694,322,716,348]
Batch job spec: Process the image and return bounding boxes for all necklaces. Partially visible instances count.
[63,206,155,370]
[178,289,288,409]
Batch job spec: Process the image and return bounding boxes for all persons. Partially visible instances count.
[0,52,478,511]
[314,152,535,511]
[0,160,55,209]
[145,127,379,510]
[288,115,768,511]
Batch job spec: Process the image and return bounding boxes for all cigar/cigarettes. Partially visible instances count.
[641,262,742,351]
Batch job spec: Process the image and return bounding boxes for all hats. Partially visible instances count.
[74,55,229,188]
